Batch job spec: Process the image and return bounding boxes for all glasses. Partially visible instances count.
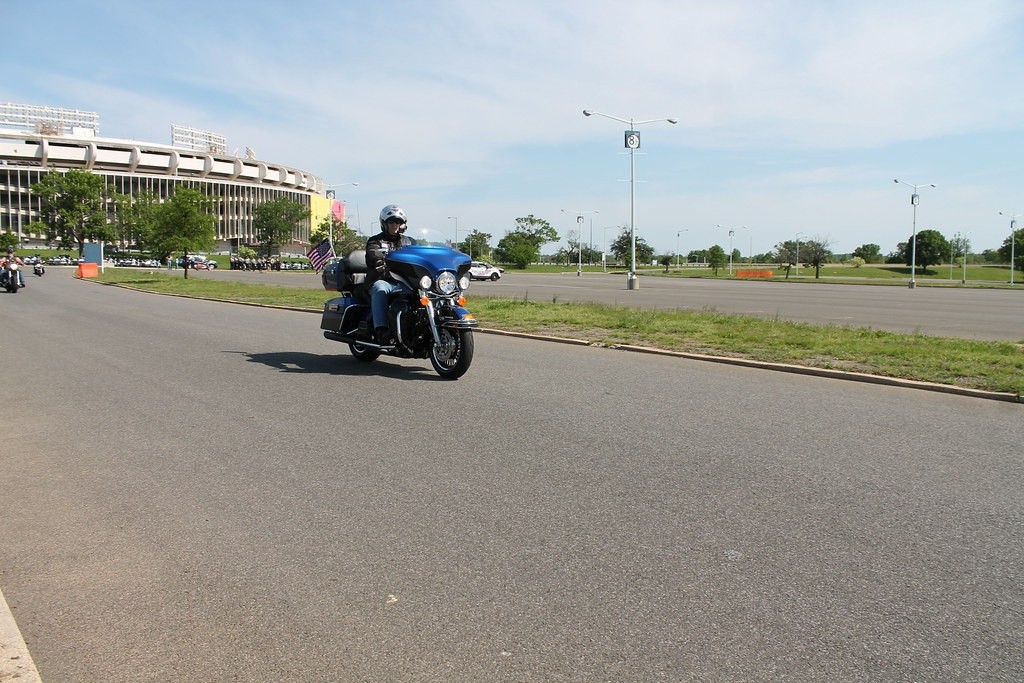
[8,252,12,254]
[387,218,401,224]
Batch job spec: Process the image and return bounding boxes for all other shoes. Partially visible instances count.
[378,329,391,343]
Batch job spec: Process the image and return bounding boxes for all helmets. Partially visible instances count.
[6,247,15,254]
[379,204,408,233]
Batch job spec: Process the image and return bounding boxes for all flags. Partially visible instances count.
[306,238,334,274]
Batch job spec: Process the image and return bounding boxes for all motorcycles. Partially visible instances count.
[320,227,479,379]
[31,258,46,278]
[0,257,27,293]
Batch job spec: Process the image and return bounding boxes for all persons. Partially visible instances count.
[34,255,45,273]
[167,256,172,270]
[0,248,26,287]
[230,256,271,273]
[175,257,179,269]
[364,204,417,346]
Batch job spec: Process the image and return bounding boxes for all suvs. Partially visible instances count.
[178,254,219,270]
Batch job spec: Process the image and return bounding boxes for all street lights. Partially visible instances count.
[677,228,689,269]
[717,224,748,275]
[796,231,808,276]
[894,178,938,289]
[448,216,457,249]
[998,211,1024,285]
[582,109,678,290]
[457,229,472,259]
[561,208,600,276]
[301,180,360,265]
[603,226,621,272]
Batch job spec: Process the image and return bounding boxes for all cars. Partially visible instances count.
[468,261,504,282]
